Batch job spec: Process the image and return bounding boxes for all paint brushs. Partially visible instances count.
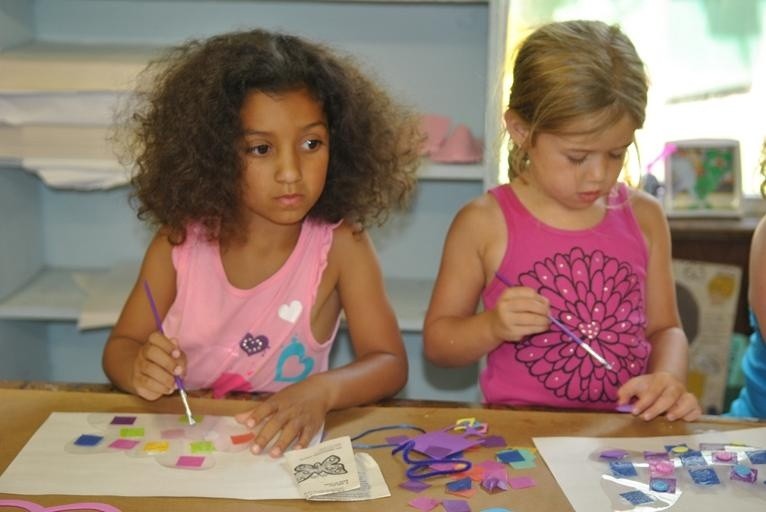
[143,280,196,425]
[495,271,612,370]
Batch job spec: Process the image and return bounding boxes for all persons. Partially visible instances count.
[420,18,706,428]
[725,138,766,424]
[100,26,409,458]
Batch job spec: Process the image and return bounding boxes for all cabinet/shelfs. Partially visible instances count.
[0,0,509,404]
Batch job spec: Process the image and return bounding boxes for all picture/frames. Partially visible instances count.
[663,138,743,220]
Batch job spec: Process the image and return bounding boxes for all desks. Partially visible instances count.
[0,380,766,512]
[667,195,766,333]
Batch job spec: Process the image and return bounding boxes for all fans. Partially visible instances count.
[673,259,744,416]
